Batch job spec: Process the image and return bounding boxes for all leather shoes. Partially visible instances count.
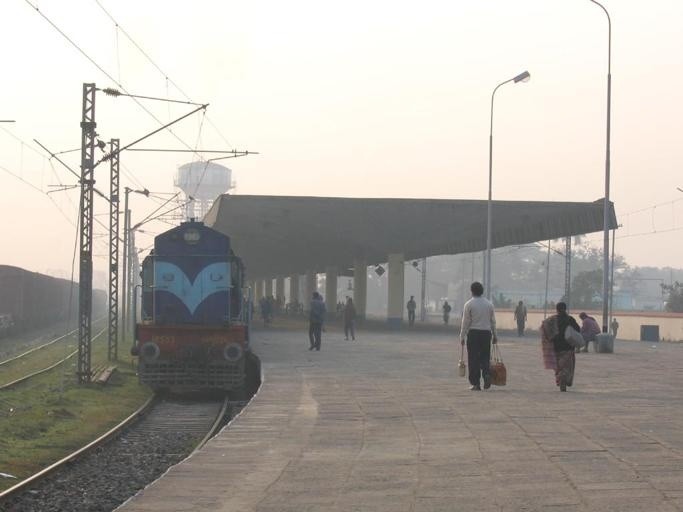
[559,375,568,392]
[485,375,491,389]
[470,384,481,391]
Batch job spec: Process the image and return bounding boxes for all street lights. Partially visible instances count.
[483,70,532,300]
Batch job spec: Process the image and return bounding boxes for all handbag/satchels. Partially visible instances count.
[564,326,585,349]
[490,360,507,386]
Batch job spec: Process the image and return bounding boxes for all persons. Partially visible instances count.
[241,291,300,326]
[406,295,415,328]
[305,291,325,351]
[342,297,355,341]
[442,300,450,325]
[513,300,528,337]
[611,317,618,339]
[458,281,497,391]
[539,302,580,394]
[573,311,601,353]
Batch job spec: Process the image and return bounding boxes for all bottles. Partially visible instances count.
[458,360,466,377]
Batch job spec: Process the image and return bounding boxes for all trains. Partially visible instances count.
[1,264,107,339]
[129,215,250,401]
